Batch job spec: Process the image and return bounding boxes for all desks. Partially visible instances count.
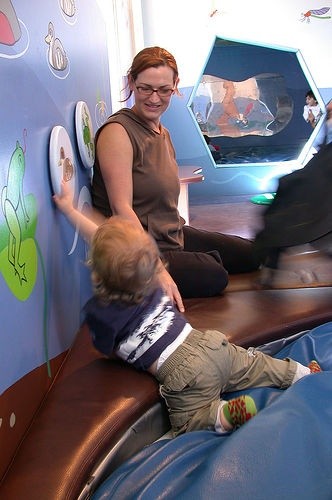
[178,165,205,227]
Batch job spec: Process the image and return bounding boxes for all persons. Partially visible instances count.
[302,90,322,127]
[53,179,322,436]
[91,47,261,297]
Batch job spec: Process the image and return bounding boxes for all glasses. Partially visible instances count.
[132,79,176,97]
